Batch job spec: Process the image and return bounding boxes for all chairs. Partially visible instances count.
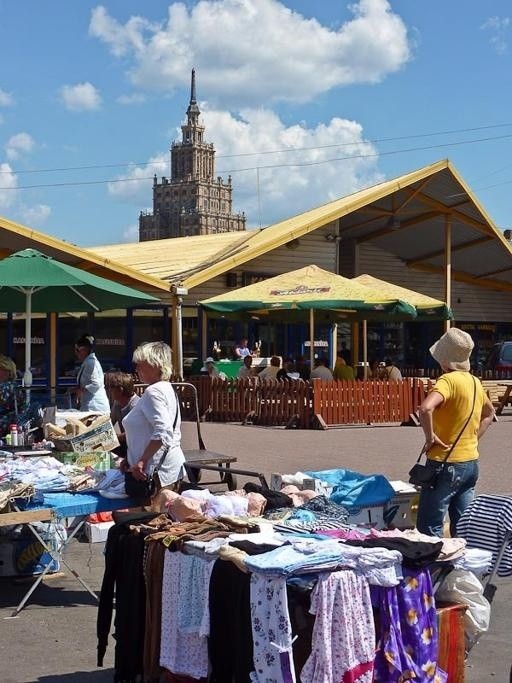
[401,377,512,422]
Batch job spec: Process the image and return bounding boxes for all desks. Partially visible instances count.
[0,491,141,617]
[115,512,469,683]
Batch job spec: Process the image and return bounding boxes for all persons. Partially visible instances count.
[73,334,111,412]
[103,369,141,458]
[199,339,403,386]
[0,353,26,447]
[416,327,496,538]
[122,340,191,512]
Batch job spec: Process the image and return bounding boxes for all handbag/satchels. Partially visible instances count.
[125,471,159,500]
[409,463,440,490]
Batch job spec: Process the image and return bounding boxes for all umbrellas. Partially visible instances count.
[0,248,161,405]
[196,265,418,372]
[349,273,454,381]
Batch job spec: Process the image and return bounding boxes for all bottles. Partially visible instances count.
[10,424,18,446]
[18,426,25,446]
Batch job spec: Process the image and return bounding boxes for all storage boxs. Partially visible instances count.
[429,599,469,683]
[348,480,420,529]
[0,539,60,577]
[84,521,115,543]
[48,415,121,453]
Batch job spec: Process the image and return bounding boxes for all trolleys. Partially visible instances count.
[130,382,238,492]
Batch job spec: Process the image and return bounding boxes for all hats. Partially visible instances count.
[429,327,475,372]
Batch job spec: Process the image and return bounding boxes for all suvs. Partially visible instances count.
[484,341,512,378]
